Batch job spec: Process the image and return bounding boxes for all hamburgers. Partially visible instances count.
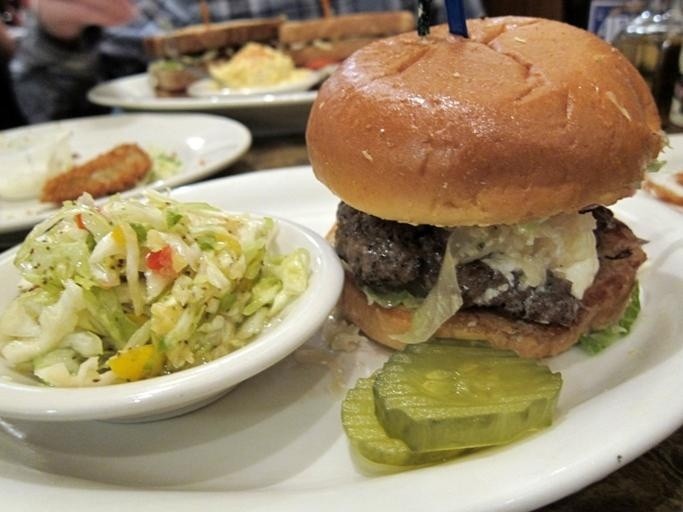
[304,14,669,359]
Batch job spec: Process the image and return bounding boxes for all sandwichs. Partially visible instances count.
[278,10,416,82]
[143,14,284,96]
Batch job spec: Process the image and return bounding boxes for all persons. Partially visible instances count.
[9,0,490,131]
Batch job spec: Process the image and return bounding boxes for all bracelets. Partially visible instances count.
[33,22,103,56]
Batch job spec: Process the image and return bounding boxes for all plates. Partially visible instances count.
[0,164,680,510]
[88,65,316,141]
[0,113,256,230]
[183,68,326,97]
[0,208,347,426]
